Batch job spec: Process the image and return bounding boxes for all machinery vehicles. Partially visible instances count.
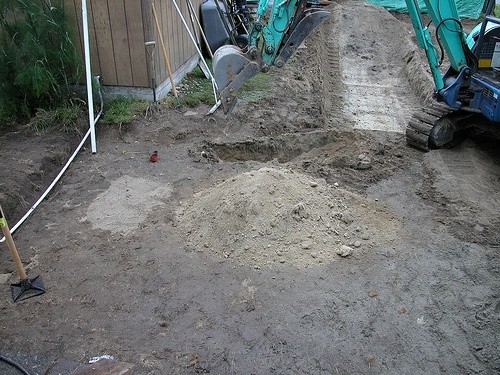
[212,0,499,151]
